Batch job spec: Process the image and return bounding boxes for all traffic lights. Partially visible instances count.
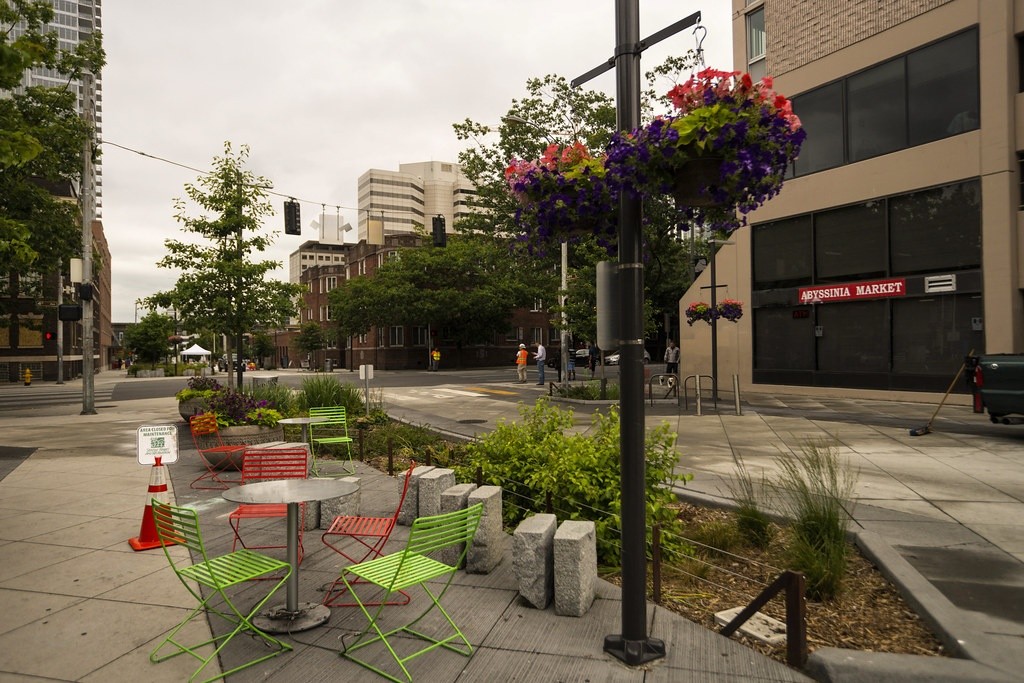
[45,330,53,344]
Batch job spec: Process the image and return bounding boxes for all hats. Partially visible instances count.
[519,344,526,348]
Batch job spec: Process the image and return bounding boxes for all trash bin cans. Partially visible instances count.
[253,376,278,391]
[325,359,333,372]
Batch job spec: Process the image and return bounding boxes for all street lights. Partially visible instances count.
[705,238,737,400]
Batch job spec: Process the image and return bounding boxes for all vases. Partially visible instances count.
[557,215,595,240]
[178,397,209,421]
[666,152,729,208]
[195,425,284,471]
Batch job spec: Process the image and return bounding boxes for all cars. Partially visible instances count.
[547,348,601,369]
[604,347,650,366]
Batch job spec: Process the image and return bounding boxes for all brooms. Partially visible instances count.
[909,348,975,437]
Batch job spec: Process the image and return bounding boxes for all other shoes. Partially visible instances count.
[516,380,526,384]
[536,383,544,386]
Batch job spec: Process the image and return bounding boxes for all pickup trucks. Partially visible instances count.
[218,353,246,372]
[965,354,1024,425]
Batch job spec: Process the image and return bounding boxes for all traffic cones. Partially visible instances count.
[127,454,186,551]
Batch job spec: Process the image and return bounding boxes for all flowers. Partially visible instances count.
[686,300,743,324]
[602,68,807,242]
[175,375,222,403]
[192,386,284,433]
[506,143,653,258]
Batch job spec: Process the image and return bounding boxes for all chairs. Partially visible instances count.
[321,461,415,607]
[341,502,483,683]
[190,415,250,491]
[228,448,307,581]
[309,405,355,478]
[149,497,294,683]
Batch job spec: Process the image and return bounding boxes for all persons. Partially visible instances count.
[568,343,576,381]
[664,341,680,373]
[531,341,546,385]
[554,347,561,382]
[118,357,130,368]
[431,346,441,372]
[515,343,528,382]
[588,340,597,379]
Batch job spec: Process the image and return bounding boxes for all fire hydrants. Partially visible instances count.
[22,368,33,386]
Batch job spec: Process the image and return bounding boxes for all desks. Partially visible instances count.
[222,479,360,633]
[277,417,329,443]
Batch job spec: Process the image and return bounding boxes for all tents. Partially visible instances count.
[181,344,212,368]
